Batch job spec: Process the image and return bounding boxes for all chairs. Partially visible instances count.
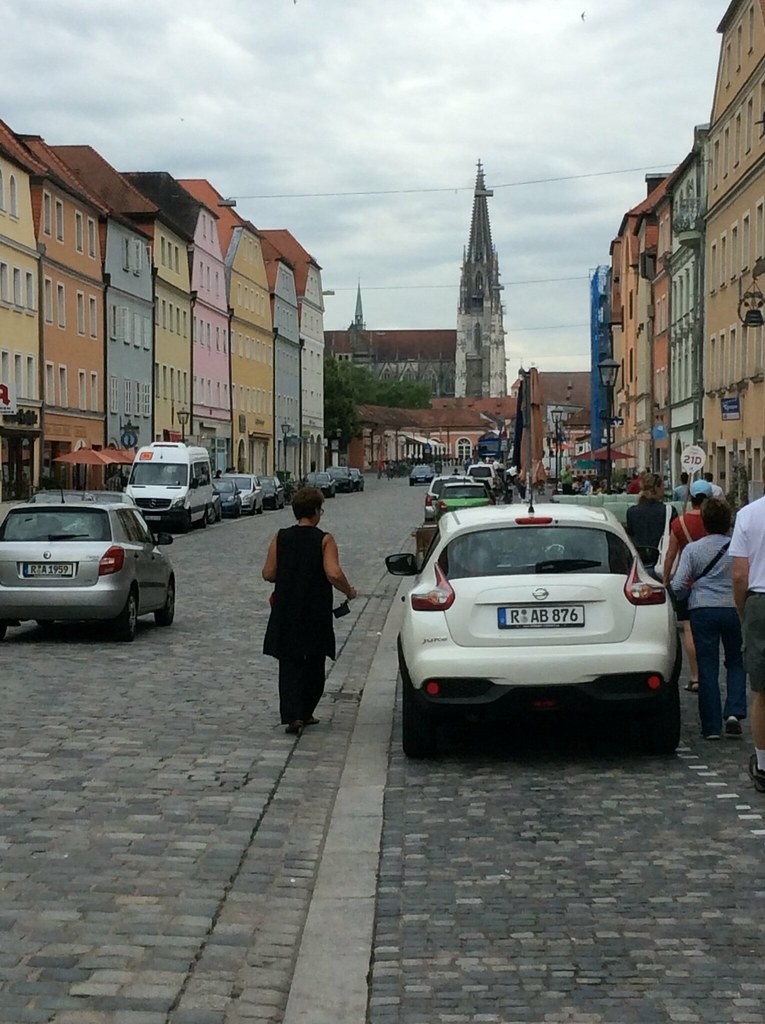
[28,517,60,538]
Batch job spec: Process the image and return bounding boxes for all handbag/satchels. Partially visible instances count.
[677,577,693,601]
[652,504,680,581]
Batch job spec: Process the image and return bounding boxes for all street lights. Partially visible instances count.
[176,407,190,442]
[597,359,622,494]
[282,420,291,482]
[551,406,564,493]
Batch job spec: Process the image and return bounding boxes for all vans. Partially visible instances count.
[124,442,212,535]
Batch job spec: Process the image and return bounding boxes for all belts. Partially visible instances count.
[744,591,765,601]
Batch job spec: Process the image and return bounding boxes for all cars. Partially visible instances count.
[222,473,265,517]
[384,505,682,758]
[212,478,242,519]
[0,504,176,642]
[256,476,285,510]
[409,466,433,486]
[90,492,134,505]
[30,491,96,503]
[424,464,503,522]
[430,482,494,523]
[349,468,364,491]
[303,471,336,498]
[327,466,353,494]
[212,484,221,523]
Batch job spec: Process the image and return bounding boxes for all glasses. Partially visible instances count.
[314,506,324,515]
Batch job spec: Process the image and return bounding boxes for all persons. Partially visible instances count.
[561,464,690,499]
[262,488,357,732]
[452,458,499,476]
[728,495,765,791]
[107,471,128,491]
[214,466,242,479]
[705,472,726,500]
[626,474,679,582]
[671,497,748,739]
[662,479,714,690]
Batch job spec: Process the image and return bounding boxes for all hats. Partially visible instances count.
[689,479,712,499]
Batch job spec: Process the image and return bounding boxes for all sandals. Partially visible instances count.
[684,680,699,692]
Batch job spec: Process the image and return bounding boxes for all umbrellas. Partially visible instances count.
[101,449,135,469]
[53,447,115,490]
[513,367,547,501]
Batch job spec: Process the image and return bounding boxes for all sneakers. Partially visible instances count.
[705,734,720,740]
[749,754,765,792]
[725,719,743,737]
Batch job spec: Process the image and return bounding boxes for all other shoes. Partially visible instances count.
[304,716,320,724]
[286,719,303,733]
